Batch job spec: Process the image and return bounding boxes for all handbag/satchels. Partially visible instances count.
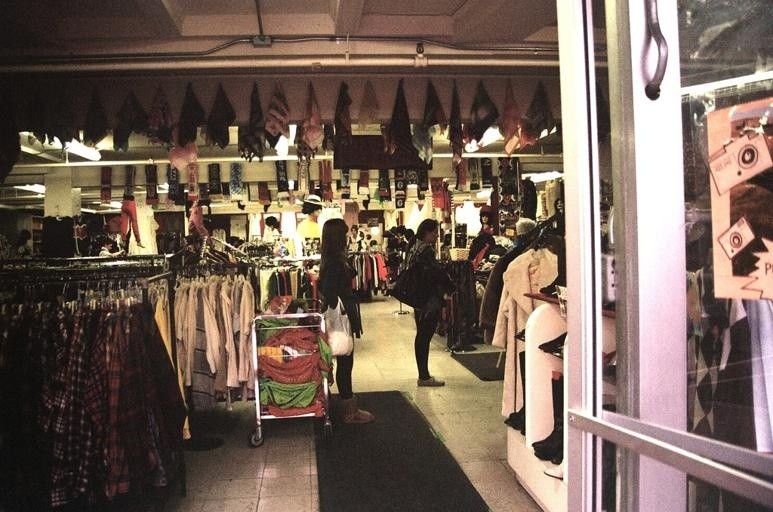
[389,268,434,308]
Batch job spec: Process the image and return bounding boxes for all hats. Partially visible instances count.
[303,195,327,208]
[516,217,537,237]
[480,205,493,225]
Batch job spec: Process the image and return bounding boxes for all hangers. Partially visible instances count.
[176,261,250,295]
[1,255,173,340]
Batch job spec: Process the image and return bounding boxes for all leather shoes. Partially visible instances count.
[539,332,566,357]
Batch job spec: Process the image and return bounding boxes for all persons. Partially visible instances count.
[120,195,145,248]
[404,217,448,387]
[263,216,280,244]
[478,205,496,235]
[298,194,323,239]
[96,239,125,259]
[344,223,378,253]
[315,216,376,426]
[186,198,214,249]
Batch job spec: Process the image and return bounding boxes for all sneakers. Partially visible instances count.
[418,376,445,386]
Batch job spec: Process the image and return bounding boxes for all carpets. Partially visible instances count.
[451,351,506,380]
[313,389,493,511]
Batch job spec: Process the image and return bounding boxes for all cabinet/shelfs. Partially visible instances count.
[252,311,333,447]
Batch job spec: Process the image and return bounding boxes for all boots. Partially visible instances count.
[532,376,564,480]
[540,248,566,293]
[336,394,374,425]
[505,351,525,435]
